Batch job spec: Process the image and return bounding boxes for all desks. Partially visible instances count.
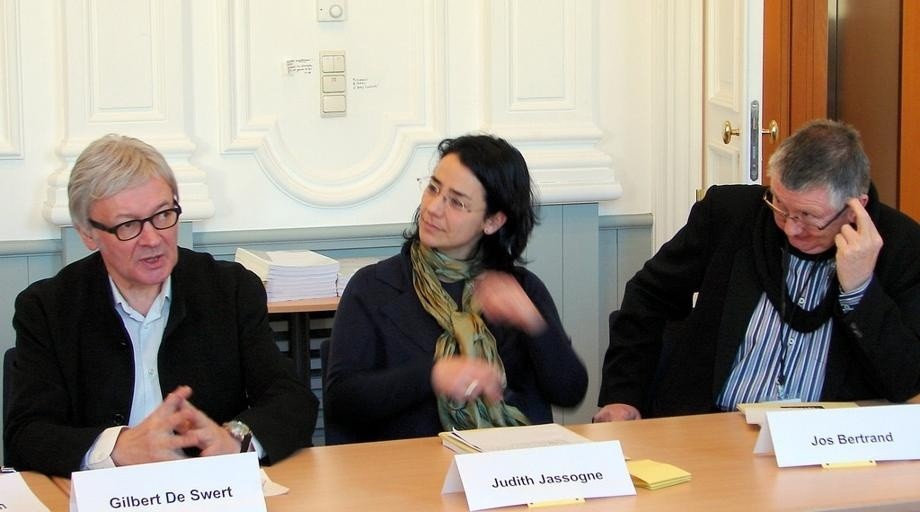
[7,395,920,512]
[266,295,344,390]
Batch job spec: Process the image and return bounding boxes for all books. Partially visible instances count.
[40,467,291,498]
[436,422,599,459]
[234,248,395,304]
[734,399,866,428]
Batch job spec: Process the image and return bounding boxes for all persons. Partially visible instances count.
[3,134,320,478]
[593,121,920,422]
[321,134,587,446]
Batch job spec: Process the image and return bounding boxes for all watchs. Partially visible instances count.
[222,419,253,453]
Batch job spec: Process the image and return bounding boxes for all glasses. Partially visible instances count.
[88,199,182,242]
[417,177,488,213]
[764,189,848,230]
